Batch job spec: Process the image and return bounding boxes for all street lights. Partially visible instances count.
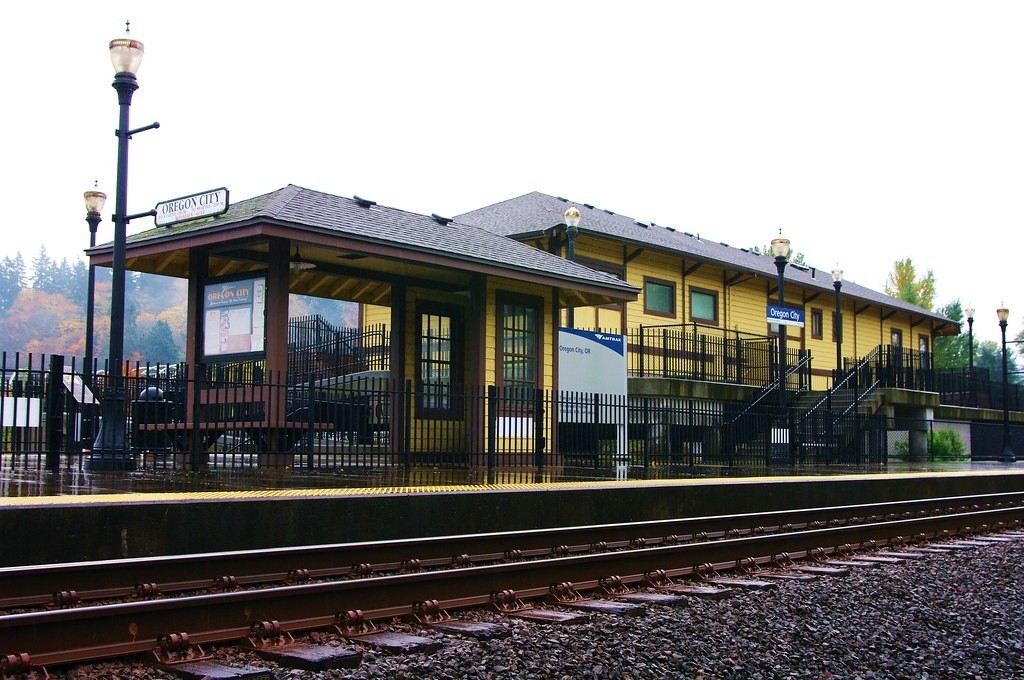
[996,302,1016,461]
[771,228,789,410]
[85,18,157,472]
[831,263,844,386]
[563,204,580,328]
[965,303,976,407]
[84,181,106,386]
